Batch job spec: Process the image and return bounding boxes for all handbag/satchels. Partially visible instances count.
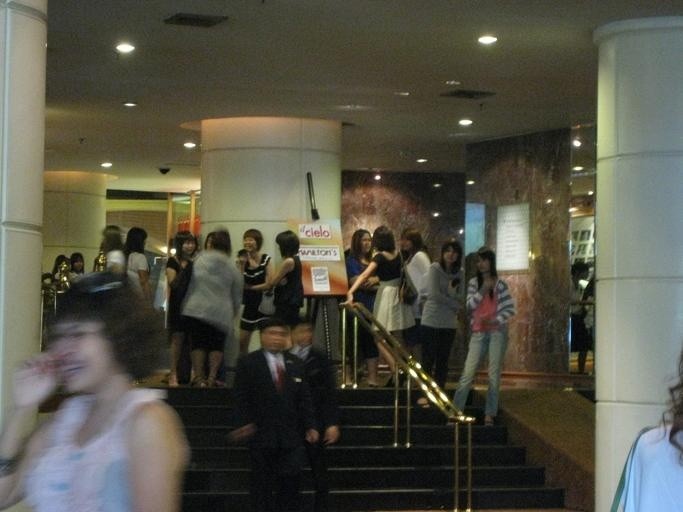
[398,269,417,306]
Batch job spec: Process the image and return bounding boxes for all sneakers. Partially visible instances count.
[190,377,227,389]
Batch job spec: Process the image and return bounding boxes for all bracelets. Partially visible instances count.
[0,459,19,476]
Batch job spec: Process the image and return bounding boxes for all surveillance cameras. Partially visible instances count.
[159,168,169,174]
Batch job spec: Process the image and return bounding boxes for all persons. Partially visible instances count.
[609,351,683,512]
[583,278,594,349]
[291,317,341,511]
[0,270,191,512]
[233,318,321,511]
[54,225,303,386]
[344,227,518,427]
[570,262,593,372]
